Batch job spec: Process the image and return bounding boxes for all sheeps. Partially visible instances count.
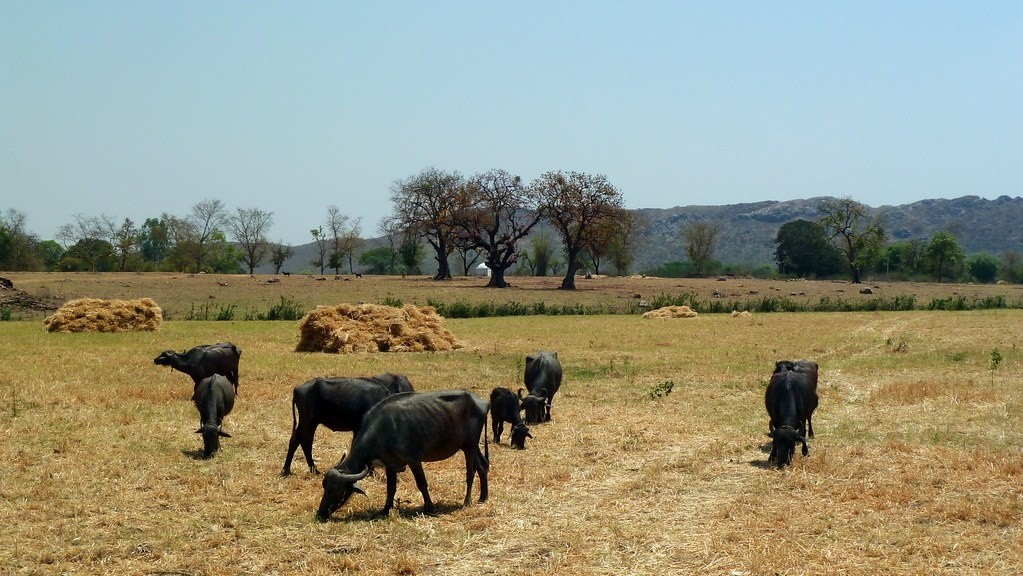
[765,359,820,470]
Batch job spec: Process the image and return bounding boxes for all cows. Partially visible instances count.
[489,387,533,450]
[517,351,562,426]
[276,370,415,478]
[191,373,235,459]
[154,342,242,401]
[315,388,501,523]
[250,271,362,285]
